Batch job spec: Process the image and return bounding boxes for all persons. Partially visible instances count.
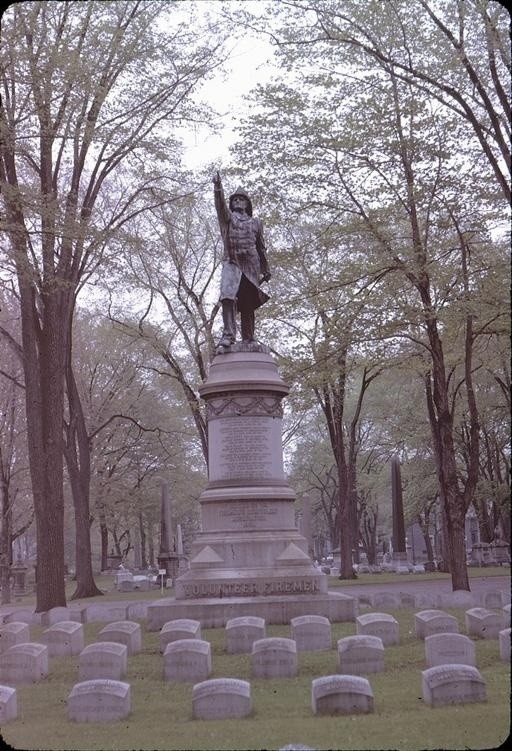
[210,169,272,351]
